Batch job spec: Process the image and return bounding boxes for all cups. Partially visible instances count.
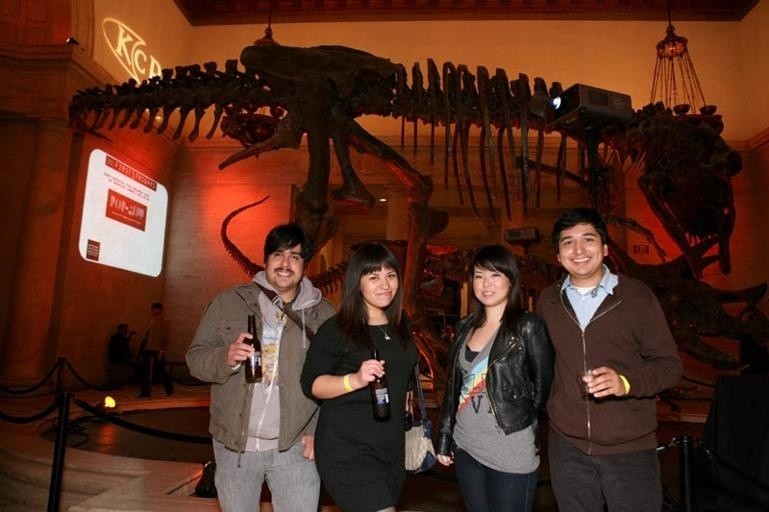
[576,368,595,400]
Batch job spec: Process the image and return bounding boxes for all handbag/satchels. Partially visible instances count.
[403,421,438,475]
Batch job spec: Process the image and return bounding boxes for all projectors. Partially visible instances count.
[545,83,633,130]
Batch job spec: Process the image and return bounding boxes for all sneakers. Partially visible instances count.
[138,381,175,397]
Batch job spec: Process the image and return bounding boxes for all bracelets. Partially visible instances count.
[344,374,354,392]
[619,374,630,397]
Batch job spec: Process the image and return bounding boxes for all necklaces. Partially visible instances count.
[376,322,391,340]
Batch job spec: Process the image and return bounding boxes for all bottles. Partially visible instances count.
[245,314,263,383]
[369,348,392,422]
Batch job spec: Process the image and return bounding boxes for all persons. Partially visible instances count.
[301,243,418,512]
[108,302,174,398]
[536,208,683,512]
[185,225,336,512]
[436,245,556,512]
